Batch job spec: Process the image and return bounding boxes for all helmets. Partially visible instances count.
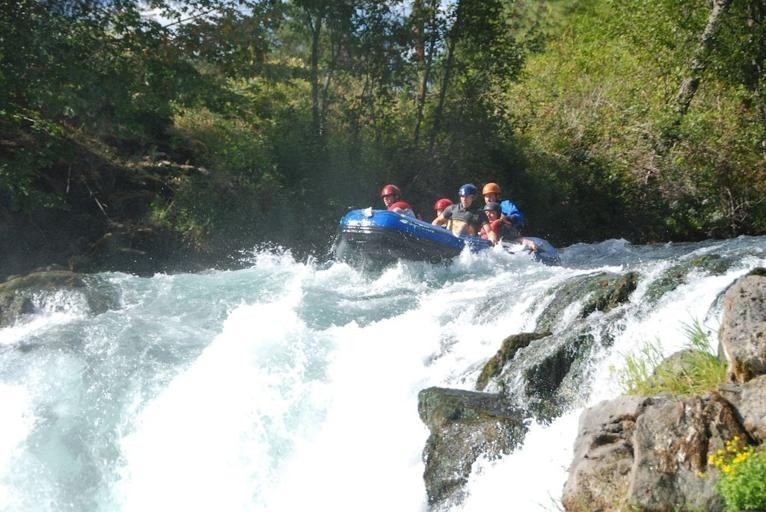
[457,184,480,197]
[381,183,401,197]
[483,202,502,220]
[435,198,454,210]
[482,182,502,195]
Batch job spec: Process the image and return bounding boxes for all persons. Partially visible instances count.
[434,198,455,227]
[431,183,498,247]
[479,202,517,243]
[380,184,417,220]
[482,181,526,229]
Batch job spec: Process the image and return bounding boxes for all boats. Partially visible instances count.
[339,210,558,264]
